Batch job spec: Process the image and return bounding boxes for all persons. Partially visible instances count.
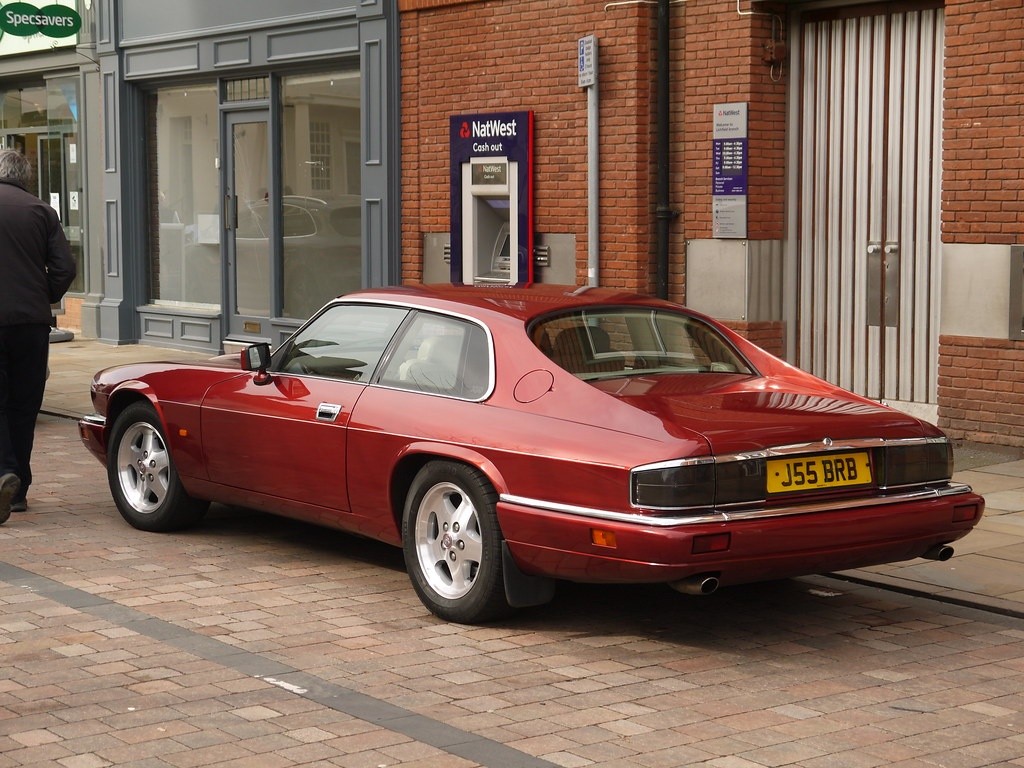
[0,150,75,526]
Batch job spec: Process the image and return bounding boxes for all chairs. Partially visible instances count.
[548,327,628,376]
[404,335,466,387]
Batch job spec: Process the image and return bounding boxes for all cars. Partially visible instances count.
[77,284,986,626]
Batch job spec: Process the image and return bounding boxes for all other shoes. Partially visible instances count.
[11,497,27,511]
[0,473,21,524]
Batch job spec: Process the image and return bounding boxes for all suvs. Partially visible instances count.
[184,188,361,303]
